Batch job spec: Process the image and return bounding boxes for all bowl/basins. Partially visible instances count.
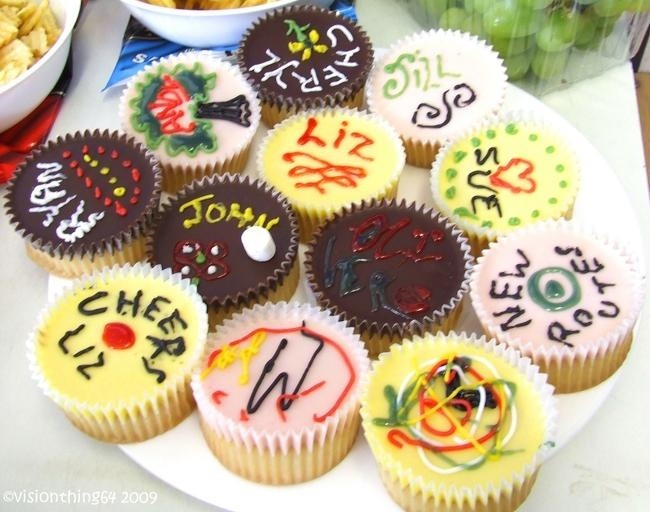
[0,0,81,134]
[122,0,298,50]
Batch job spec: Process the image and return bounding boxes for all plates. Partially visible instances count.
[47,45,649,511]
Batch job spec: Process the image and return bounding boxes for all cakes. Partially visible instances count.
[4,6,642,512]
